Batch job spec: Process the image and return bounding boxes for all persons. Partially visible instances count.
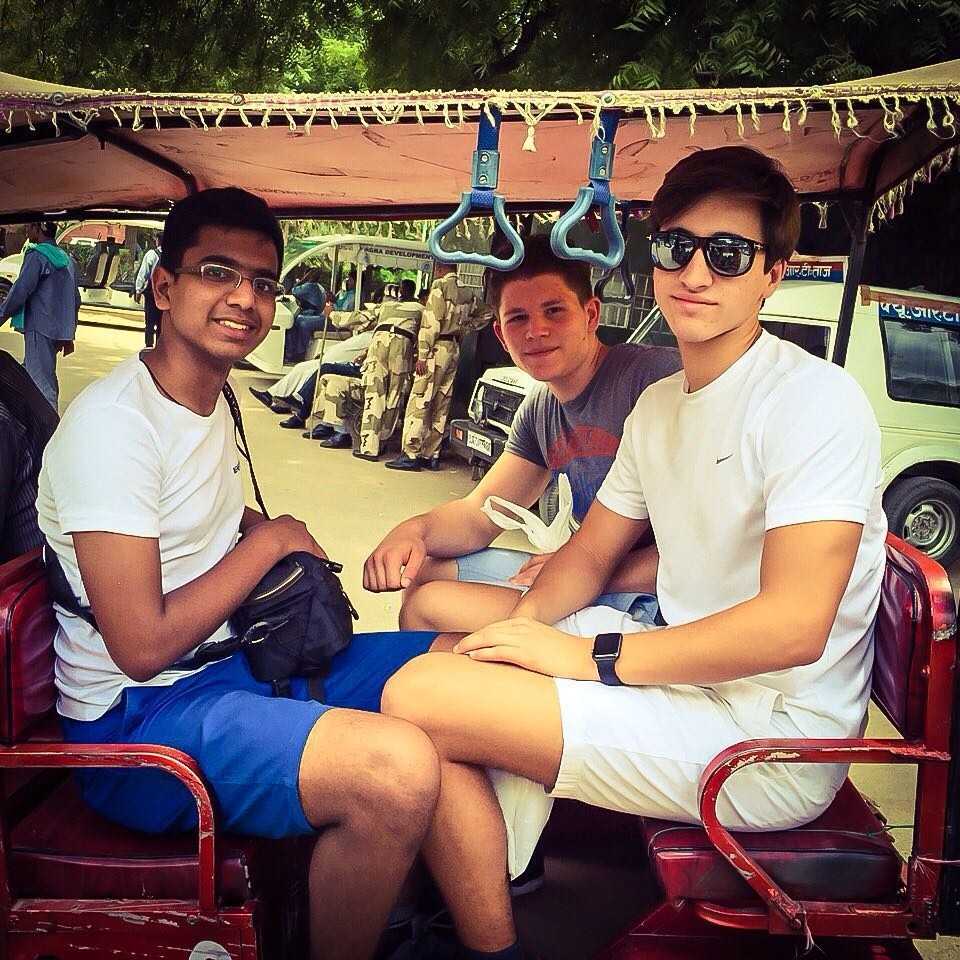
[380,146,888,960]
[292,267,327,315]
[249,330,373,449]
[395,279,418,302]
[384,262,494,472]
[36,187,468,960]
[0,222,81,413]
[362,233,667,632]
[135,231,163,349]
[284,270,364,363]
[323,300,425,463]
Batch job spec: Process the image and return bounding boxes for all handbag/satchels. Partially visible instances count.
[228,553,356,683]
[480,472,574,555]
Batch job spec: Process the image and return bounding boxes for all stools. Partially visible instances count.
[313,331,352,340]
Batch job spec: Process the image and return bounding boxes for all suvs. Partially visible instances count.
[448,282,960,565]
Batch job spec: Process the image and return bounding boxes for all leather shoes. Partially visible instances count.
[269,405,292,414]
[248,386,272,407]
[303,424,334,440]
[320,432,353,447]
[385,455,419,471]
[274,395,302,412]
[280,415,307,429]
[419,456,439,471]
[353,449,378,462]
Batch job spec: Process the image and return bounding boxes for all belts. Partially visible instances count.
[436,334,461,343]
[374,324,414,342]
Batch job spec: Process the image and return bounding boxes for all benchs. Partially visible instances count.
[637,529,956,940]
[0,548,261,908]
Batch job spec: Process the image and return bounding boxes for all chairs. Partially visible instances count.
[105,245,135,291]
[78,241,107,288]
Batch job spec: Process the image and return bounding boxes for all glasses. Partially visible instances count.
[173,261,285,304]
[646,229,765,279]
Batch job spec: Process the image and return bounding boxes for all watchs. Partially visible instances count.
[592,633,623,685]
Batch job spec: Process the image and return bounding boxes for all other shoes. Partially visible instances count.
[506,855,544,896]
